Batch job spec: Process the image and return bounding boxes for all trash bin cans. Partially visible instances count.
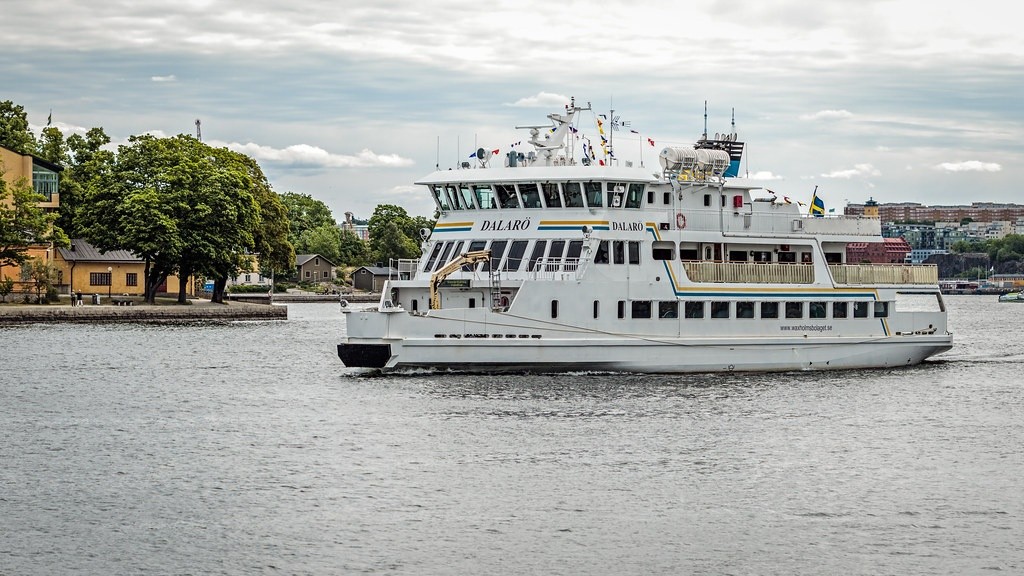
[92,293,100,305]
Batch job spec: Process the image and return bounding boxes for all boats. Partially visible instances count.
[998,290,1024,302]
[335,95,955,373]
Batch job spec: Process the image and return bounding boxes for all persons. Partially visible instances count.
[77,289,83,306]
[491,197,497,209]
[594,187,602,204]
[71,289,75,307]
[470,197,475,209]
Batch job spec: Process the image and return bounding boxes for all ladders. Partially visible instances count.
[492,269,502,306]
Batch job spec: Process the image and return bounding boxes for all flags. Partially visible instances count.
[810,195,825,218]
[569,114,654,165]
[468,127,558,156]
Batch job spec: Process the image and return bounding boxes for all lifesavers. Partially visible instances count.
[677,212,685,229]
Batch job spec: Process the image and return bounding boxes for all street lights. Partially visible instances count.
[108,267,113,298]
